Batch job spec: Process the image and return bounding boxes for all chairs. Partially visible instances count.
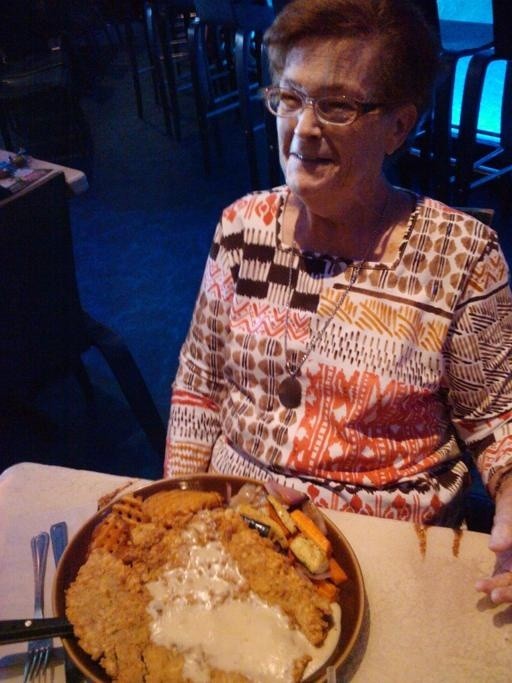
[0,0,512,470]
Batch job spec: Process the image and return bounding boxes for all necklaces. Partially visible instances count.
[283,182,394,408]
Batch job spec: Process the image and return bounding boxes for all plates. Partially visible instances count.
[54,472,363,682]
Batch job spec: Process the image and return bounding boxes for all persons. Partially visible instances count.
[163,0,512,604]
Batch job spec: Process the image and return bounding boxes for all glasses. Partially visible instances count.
[261,86,389,128]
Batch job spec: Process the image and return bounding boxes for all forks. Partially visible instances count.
[22,532,52,683]
